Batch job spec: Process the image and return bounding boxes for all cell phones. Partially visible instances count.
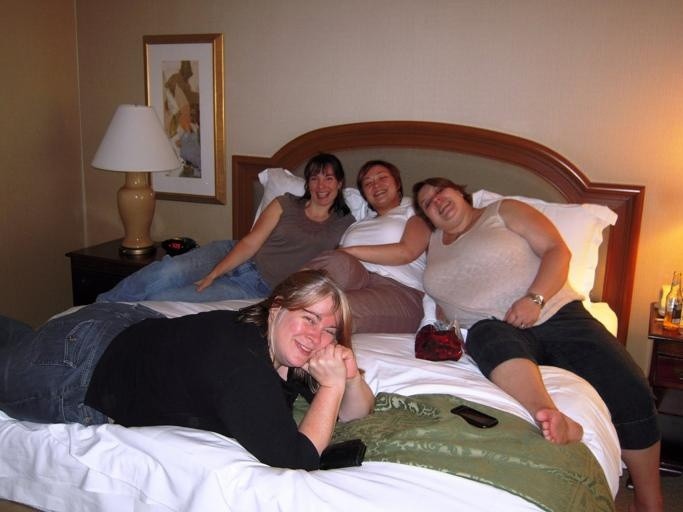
[450,404,498,429]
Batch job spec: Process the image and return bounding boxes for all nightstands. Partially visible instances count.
[64,236,177,309]
[645,299,682,491]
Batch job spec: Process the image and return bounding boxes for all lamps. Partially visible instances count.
[91,100,180,251]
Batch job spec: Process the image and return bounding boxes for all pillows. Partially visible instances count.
[342,187,413,222]
[472,189,619,300]
[252,169,306,234]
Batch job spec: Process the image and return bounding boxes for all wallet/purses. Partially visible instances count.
[319,438,367,468]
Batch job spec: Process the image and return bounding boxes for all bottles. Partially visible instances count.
[662,270,683,332]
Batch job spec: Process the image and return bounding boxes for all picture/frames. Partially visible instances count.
[143,30,228,207]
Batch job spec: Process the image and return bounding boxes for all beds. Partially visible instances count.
[1,116,648,511]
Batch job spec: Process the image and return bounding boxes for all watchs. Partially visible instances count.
[527,291,546,309]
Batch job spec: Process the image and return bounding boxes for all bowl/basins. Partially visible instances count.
[161,237,197,257]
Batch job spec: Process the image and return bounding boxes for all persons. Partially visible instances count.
[298,159,432,335]
[0,268,376,472]
[95,152,356,305]
[411,176,665,511]
